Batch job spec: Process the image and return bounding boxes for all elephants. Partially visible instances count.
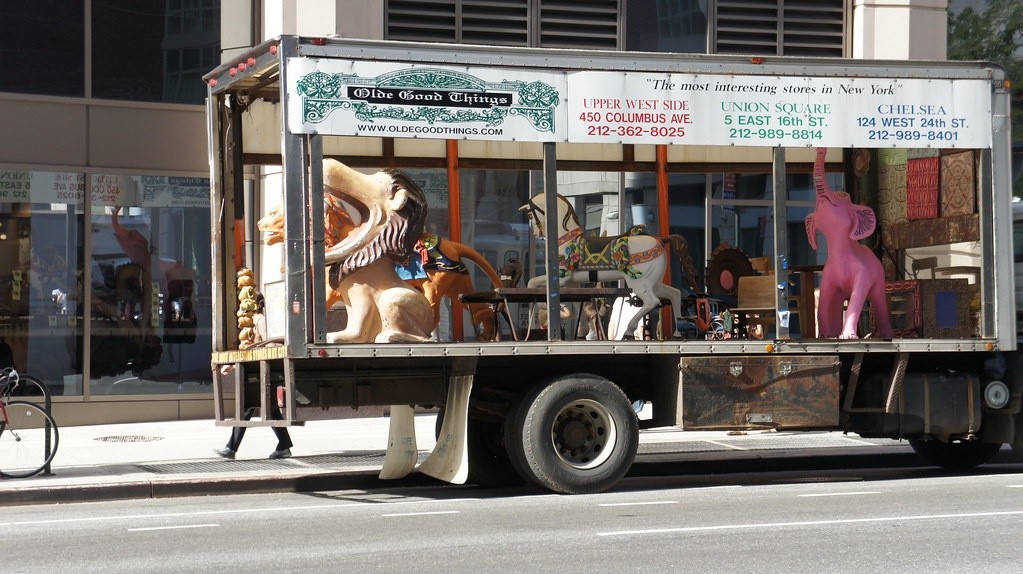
[805,147,896,339]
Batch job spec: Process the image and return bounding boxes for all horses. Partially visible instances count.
[517,192,697,341]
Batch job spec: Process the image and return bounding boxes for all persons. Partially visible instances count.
[215,291,293,459]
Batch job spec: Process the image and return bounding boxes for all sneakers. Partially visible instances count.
[269,447,291,458]
[213,448,236,460]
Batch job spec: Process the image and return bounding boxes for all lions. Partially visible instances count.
[257,158,507,341]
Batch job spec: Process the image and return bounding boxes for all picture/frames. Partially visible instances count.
[917,278,972,340]
[867,278,923,342]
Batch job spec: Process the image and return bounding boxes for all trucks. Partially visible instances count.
[199,21,1019,499]
